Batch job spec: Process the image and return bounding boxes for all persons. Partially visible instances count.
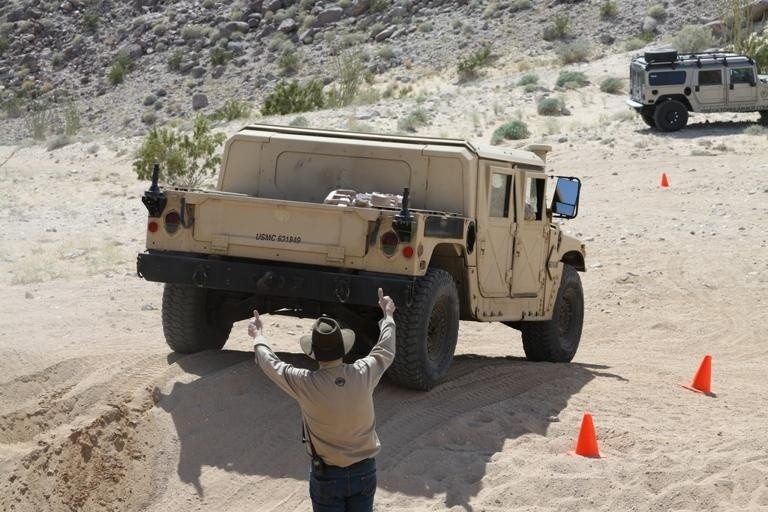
[246,287,398,512]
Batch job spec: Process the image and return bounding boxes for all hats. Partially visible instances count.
[300,317,356,362]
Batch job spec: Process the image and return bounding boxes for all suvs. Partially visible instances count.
[143,120,590,393]
[627,45,768,132]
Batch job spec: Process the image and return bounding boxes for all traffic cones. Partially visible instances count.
[657,173,669,188]
[574,412,605,461]
[680,354,714,396]
